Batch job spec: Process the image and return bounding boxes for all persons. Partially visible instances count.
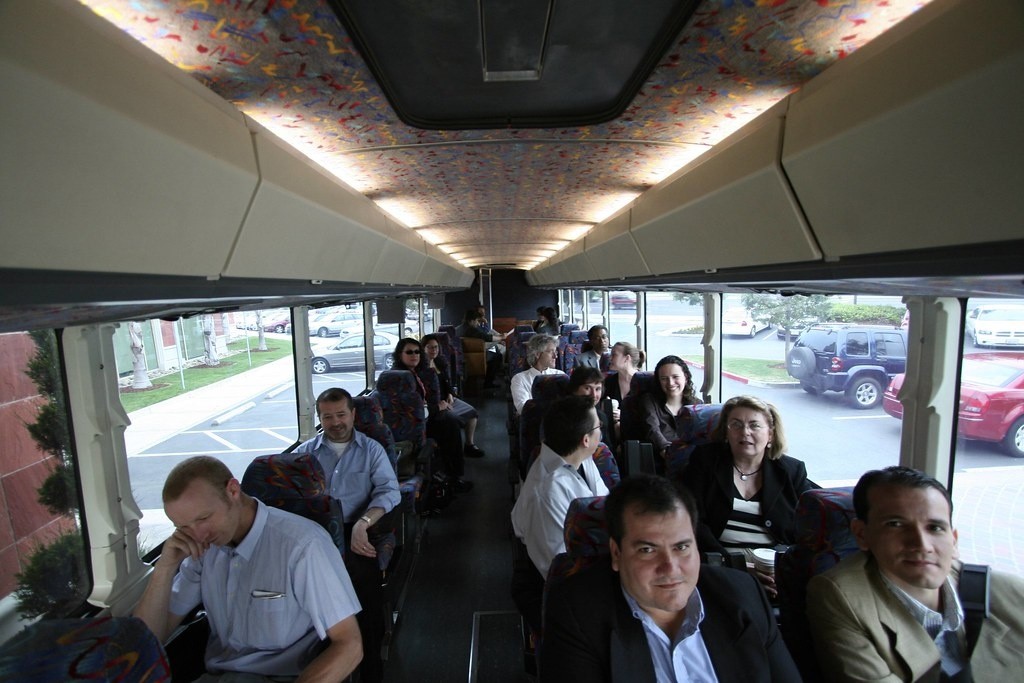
[133,305,1022,683]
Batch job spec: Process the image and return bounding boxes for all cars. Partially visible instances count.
[967,303,1024,349]
[338,318,420,339]
[234,306,378,337]
[777,317,816,340]
[611,291,638,309]
[311,332,400,374]
[882,350,1023,457]
[408,303,434,324]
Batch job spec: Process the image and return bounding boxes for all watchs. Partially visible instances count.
[361,515,373,527]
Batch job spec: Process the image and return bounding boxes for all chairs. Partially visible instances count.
[0,317,867,683]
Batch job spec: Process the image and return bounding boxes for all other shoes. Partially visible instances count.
[485,382,502,389]
[464,442,485,458]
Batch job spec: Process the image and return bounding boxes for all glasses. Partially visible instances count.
[538,319,545,324]
[726,420,761,431]
[401,349,421,355]
[426,344,438,350]
[587,420,604,433]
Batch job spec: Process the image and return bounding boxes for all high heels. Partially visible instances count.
[419,498,449,520]
[437,472,476,503]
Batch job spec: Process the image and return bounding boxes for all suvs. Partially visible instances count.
[785,322,915,410]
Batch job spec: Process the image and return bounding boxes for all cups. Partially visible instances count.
[754,548,776,599]
[610,399,619,422]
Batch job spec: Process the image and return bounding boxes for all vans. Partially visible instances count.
[721,295,771,337]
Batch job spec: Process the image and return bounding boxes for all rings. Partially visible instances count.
[364,547,365,550]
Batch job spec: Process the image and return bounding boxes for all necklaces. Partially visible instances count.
[734,462,762,480]
